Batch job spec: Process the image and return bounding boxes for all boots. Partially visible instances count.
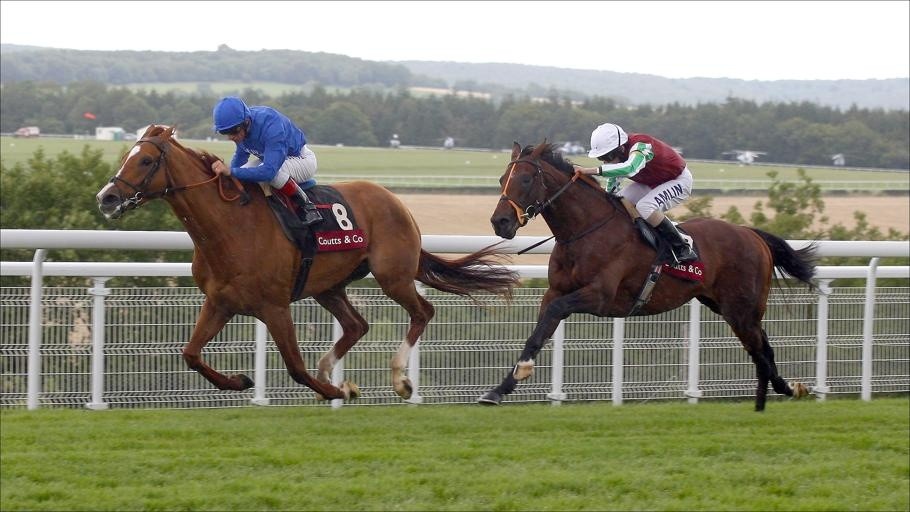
[280,177,323,226]
[654,217,697,264]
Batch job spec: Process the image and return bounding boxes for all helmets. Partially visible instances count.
[213,96,250,131]
[588,123,628,158]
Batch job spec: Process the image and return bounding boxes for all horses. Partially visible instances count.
[473,135,830,414]
[95,121,522,401]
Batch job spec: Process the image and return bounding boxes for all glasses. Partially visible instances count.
[219,126,241,135]
[597,150,617,161]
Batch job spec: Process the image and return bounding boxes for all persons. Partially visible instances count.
[209,95,324,228]
[571,122,699,266]
